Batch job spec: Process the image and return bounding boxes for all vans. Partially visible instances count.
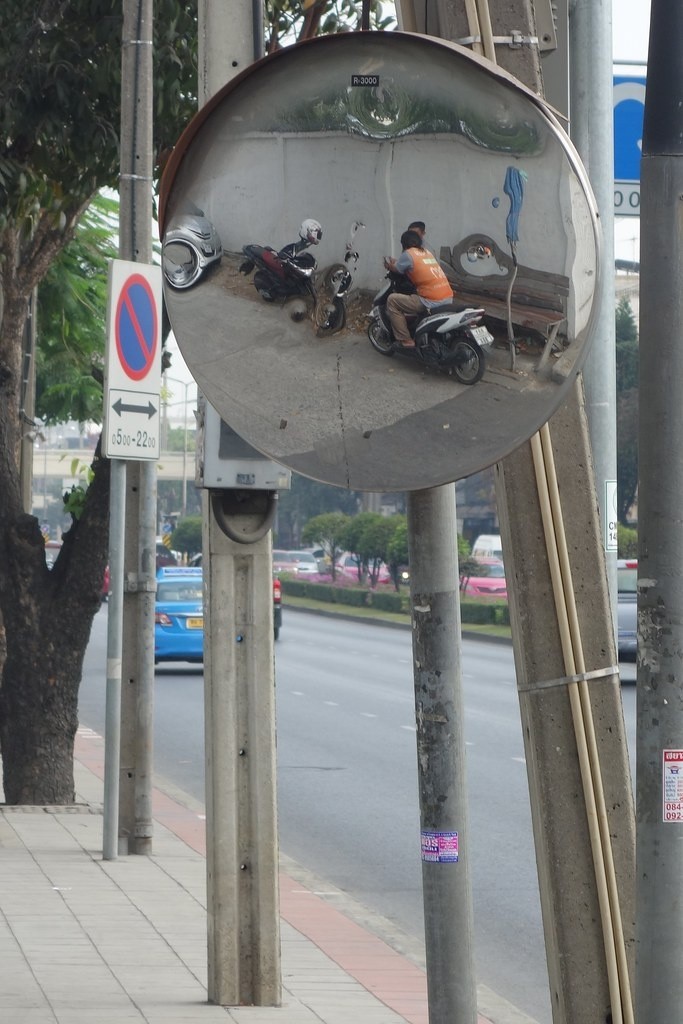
[471,534,503,557]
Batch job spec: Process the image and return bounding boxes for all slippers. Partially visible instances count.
[392,341,416,350]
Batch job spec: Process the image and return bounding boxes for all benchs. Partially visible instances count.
[435,234,568,373]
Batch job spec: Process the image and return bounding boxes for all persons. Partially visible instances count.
[379,215,456,350]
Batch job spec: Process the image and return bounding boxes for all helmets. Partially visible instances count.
[299,219,322,245]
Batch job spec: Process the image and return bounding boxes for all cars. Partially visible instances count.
[459,557,507,598]
[290,549,319,575]
[272,549,299,576]
[160,199,223,290]
[155,542,178,569]
[333,551,392,584]
[188,554,202,567]
[303,548,332,574]
[154,565,203,662]
[101,564,111,601]
[616,558,639,662]
[274,578,283,640]
[44,540,62,571]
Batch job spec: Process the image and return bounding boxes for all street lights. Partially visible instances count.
[164,374,200,518]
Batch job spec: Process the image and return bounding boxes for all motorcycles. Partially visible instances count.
[240,237,355,339]
[363,270,495,385]
